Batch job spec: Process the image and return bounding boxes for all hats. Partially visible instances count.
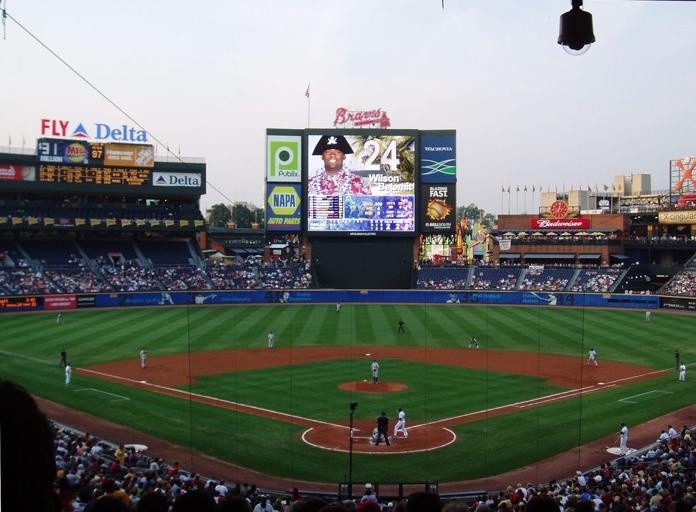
[312,135,354,154]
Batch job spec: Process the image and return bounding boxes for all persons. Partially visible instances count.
[0,379,58,512]
[335,302,341,313]
[267,331,275,350]
[60,350,71,383]
[645,310,652,321]
[588,348,596,368]
[307,134,370,197]
[469,337,479,349]
[1,191,315,293]
[675,350,686,381]
[56,311,65,326]
[375,407,408,446]
[140,349,148,367]
[372,359,380,384]
[397,319,407,334]
[49,421,695,512]
[418,232,695,299]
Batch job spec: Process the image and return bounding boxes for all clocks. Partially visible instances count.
[550,200,569,220]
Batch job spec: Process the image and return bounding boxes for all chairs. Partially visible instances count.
[211,262,309,289]
[418,249,694,292]
[0,190,210,289]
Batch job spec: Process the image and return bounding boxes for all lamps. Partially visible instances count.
[554,0,599,58]
